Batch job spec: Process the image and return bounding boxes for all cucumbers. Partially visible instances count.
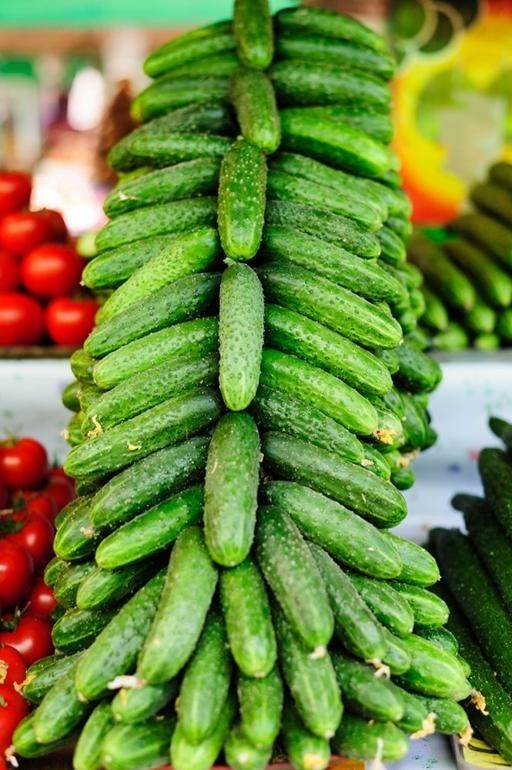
[413,160,512,355]
[421,416,512,763]
[11,0,478,770]
